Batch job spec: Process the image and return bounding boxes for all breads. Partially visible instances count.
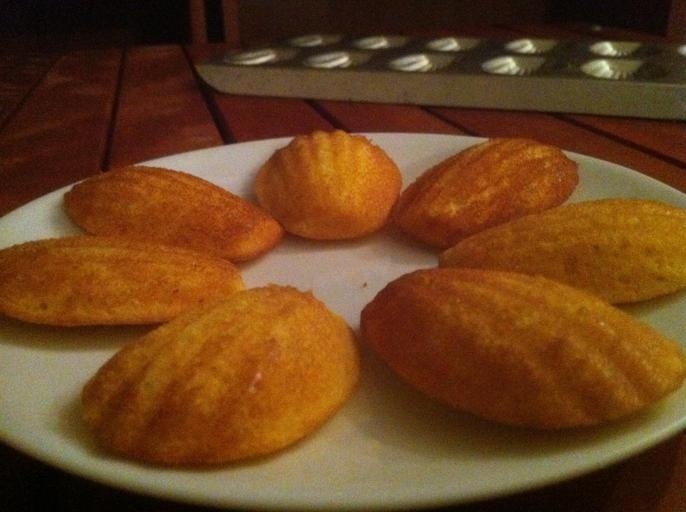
[255,129,403,240]
[360,267,686,433]
[0,235,243,327]
[439,198,686,306]
[64,165,284,263]
[392,137,579,249]
[82,283,363,465]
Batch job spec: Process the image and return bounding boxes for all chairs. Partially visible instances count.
[186,0,553,46]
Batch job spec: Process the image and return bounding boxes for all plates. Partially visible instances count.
[0,133,686,511]
